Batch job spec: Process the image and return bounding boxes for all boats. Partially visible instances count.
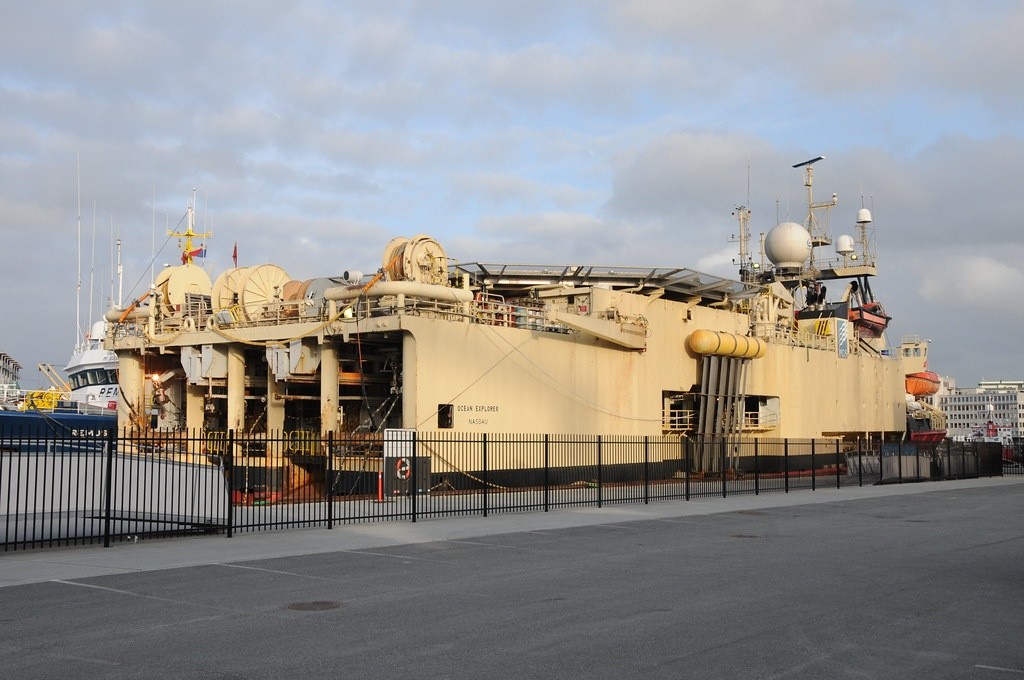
[61,155,120,416]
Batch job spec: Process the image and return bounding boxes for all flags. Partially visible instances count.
[189,248,206,258]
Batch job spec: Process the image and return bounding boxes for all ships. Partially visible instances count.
[113,154,949,512]
